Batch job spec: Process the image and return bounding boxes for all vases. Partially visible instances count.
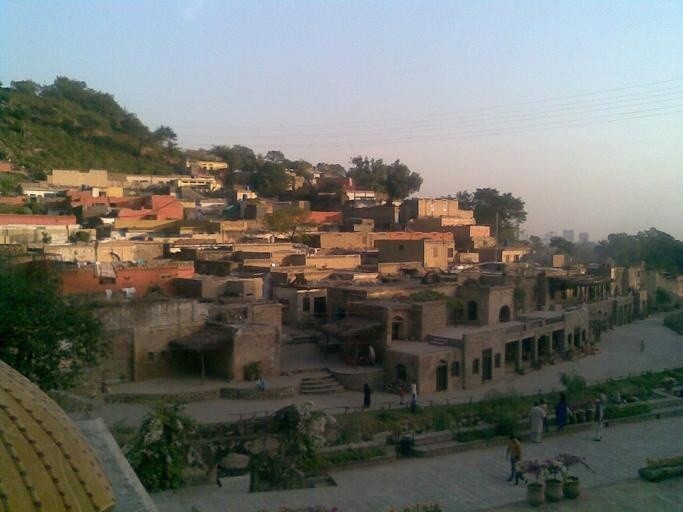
[561,471,581,501]
[540,476,563,501]
[526,480,544,505]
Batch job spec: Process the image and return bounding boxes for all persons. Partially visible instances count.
[411,393,419,415]
[638,339,646,350]
[592,399,604,441]
[367,343,377,365]
[257,375,266,391]
[512,454,526,485]
[506,433,523,481]
[539,398,548,433]
[364,383,372,408]
[552,391,568,433]
[399,384,405,405]
[411,374,419,394]
[528,401,545,443]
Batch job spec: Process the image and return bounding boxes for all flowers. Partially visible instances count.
[515,450,600,487]
